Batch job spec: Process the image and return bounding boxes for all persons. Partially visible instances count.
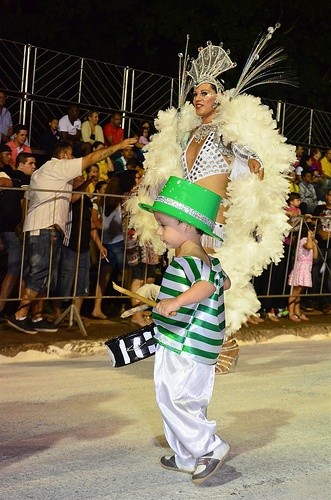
[120,23,298,375]
[138,176,231,484]
[0,93,331,335]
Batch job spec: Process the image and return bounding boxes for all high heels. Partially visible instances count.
[215,339,241,375]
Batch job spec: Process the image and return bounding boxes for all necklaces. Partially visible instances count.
[193,123,213,144]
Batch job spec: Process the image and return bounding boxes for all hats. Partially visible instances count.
[138,176,223,243]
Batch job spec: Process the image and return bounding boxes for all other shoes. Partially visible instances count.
[91,315,111,320]
[130,318,147,326]
[160,455,193,474]
[191,442,231,483]
[299,314,309,321]
[8,315,37,335]
[31,319,59,332]
[251,315,264,322]
[288,315,301,322]
[266,316,280,322]
